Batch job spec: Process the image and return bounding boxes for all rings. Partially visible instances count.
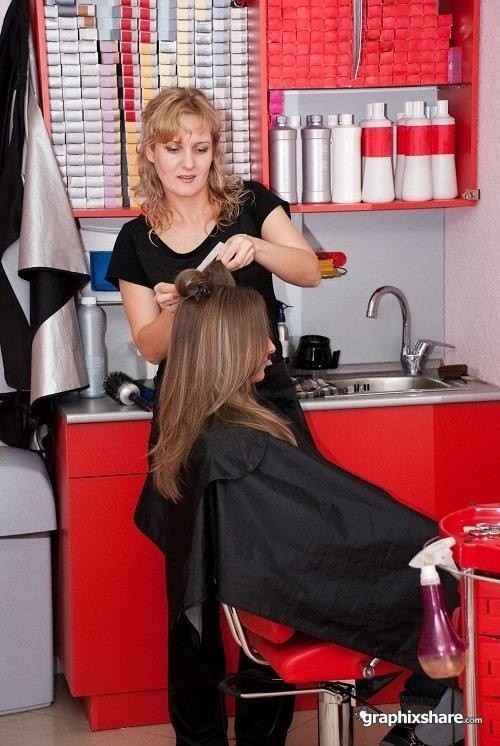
[165,300,171,304]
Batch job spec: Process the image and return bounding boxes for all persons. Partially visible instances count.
[104,83,321,746]
[148,259,467,746]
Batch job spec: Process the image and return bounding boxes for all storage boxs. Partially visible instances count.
[0,448,54,716]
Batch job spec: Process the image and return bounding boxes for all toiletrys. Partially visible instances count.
[406,534,466,682]
[276,298,293,366]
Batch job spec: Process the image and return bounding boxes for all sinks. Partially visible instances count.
[323,370,477,398]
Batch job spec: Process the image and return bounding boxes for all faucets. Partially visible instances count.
[366,286,410,367]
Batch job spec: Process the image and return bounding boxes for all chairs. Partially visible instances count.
[222,602,415,746]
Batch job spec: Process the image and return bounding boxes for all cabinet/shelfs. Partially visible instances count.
[51,401,500,732]
[441,503,500,745]
[30,0,481,218]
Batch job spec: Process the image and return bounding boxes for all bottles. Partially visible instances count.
[293,334,341,370]
[276,299,291,359]
[268,115,298,205]
[290,111,363,204]
[359,97,460,203]
[76,296,109,400]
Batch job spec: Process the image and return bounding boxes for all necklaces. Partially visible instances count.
[168,225,209,246]
